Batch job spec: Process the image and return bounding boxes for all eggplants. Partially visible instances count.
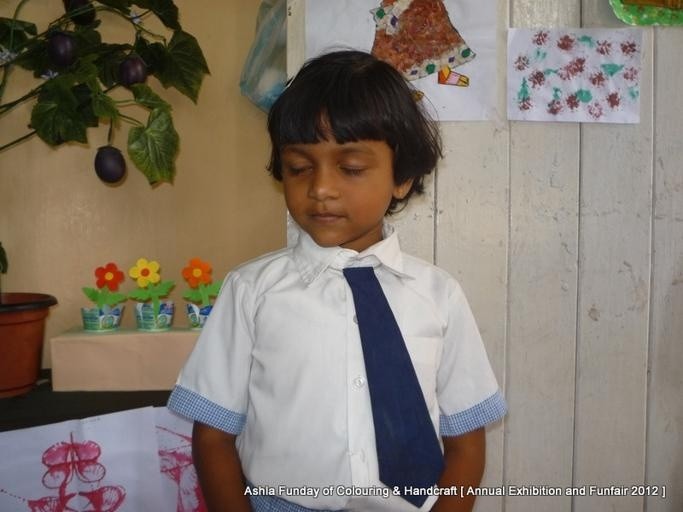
[94,116,125,184]
[46,20,75,69]
[118,30,148,88]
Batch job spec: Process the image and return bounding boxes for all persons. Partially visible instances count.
[169,50,507,512]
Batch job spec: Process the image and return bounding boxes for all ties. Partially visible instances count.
[344,265,443,507]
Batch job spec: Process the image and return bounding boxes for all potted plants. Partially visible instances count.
[1,2,210,400]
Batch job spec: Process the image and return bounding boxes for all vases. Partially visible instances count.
[134,300,174,332]
[81,304,124,333]
[183,302,213,330]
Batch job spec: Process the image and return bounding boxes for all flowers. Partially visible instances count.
[128,258,177,301]
[181,258,227,305]
[80,262,126,307]
